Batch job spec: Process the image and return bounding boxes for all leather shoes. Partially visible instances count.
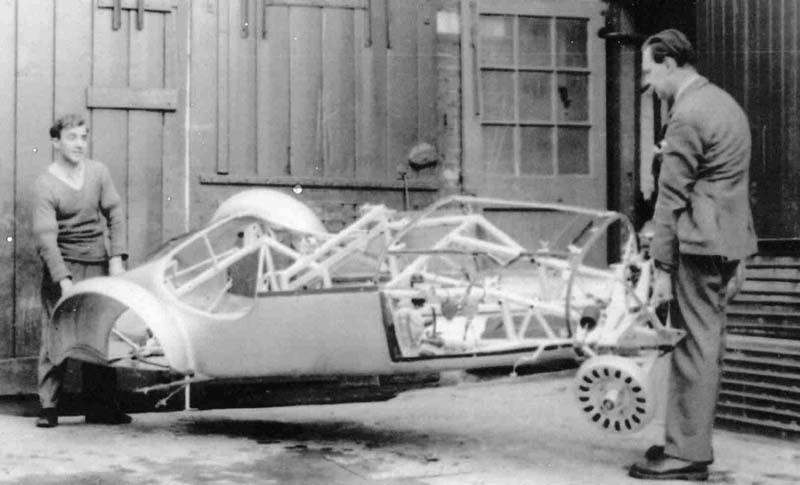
[36,413,58,428]
[628,456,709,482]
[84,400,133,425]
[645,444,715,465]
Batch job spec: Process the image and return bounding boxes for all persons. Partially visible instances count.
[31,113,133,428]
[628,25,759,483]
